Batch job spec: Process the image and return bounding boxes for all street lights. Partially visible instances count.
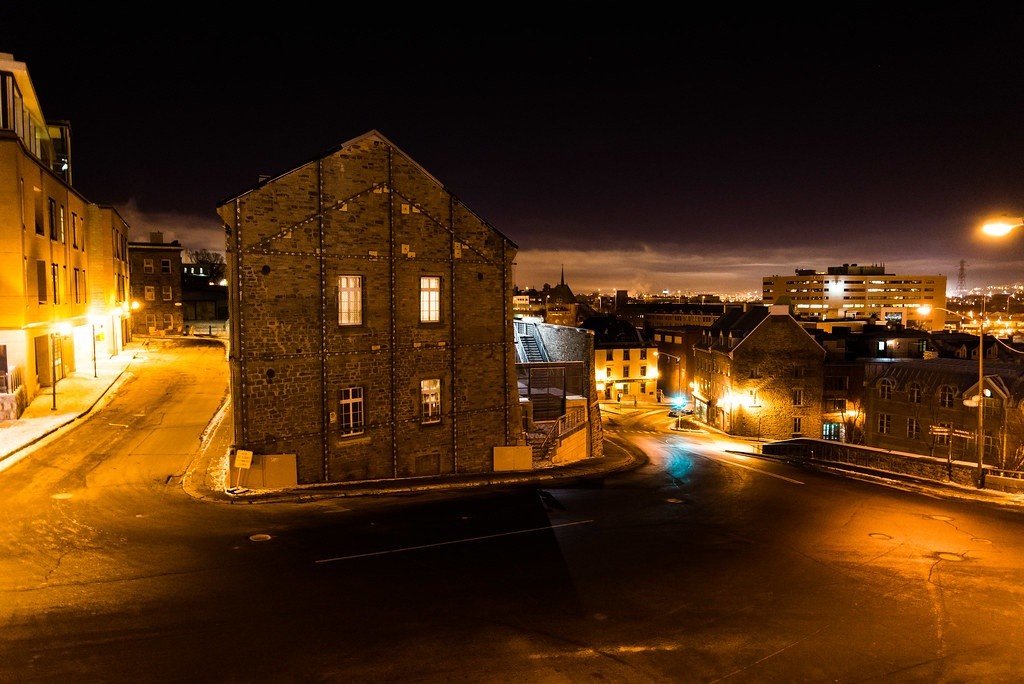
[653,352,682,416]
[89,317,104,381]
[47,321,66,413]
[674,395,685,430]
[917,301,986,487]
